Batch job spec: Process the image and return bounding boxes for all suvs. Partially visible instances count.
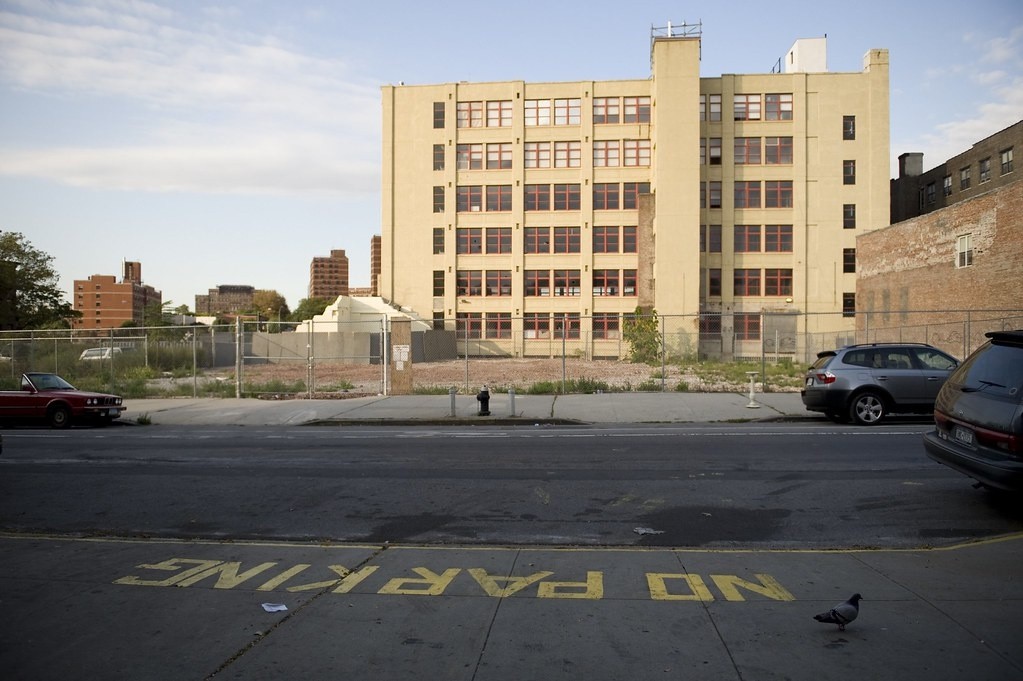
[923,330,1023,490]
[801,342,964,424]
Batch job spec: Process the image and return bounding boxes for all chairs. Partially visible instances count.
[887,359,908,369]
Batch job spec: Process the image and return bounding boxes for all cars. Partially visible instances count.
[0,372,127,430]
[79,347,122,359]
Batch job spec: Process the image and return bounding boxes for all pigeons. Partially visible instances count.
[813,592,863,631]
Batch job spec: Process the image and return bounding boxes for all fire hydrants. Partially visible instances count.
[476,385,491,416]
